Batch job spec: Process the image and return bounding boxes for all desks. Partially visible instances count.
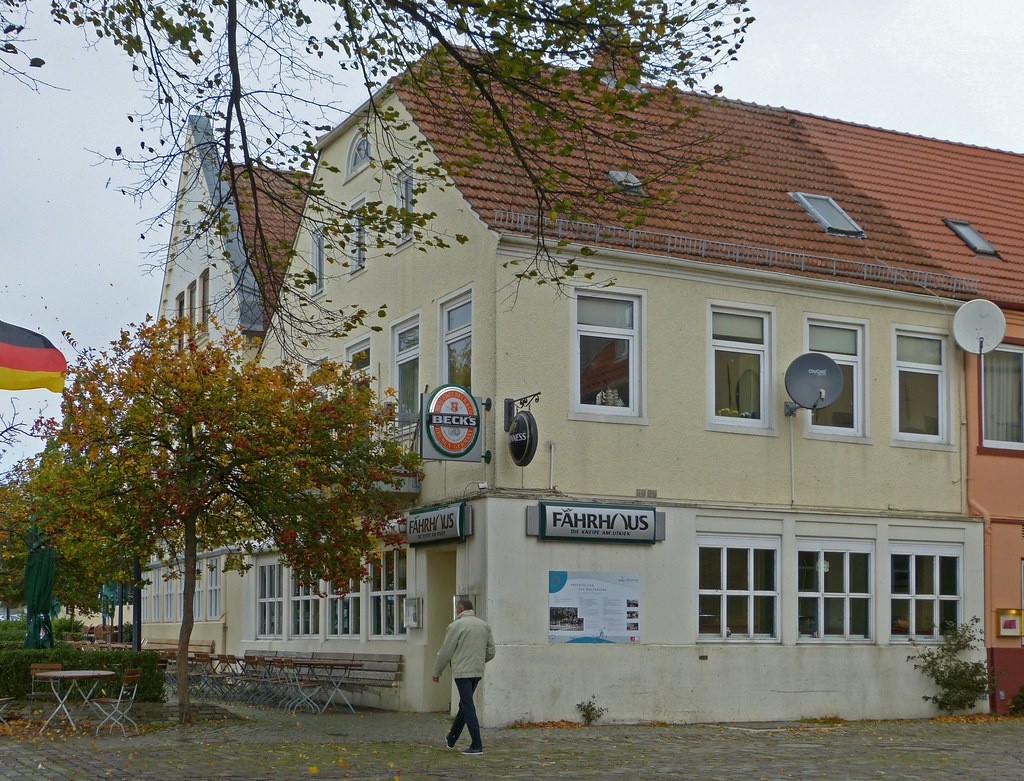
[296,662,364,715]
[35,670,116,739]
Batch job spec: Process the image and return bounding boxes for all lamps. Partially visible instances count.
[462,480,489,506]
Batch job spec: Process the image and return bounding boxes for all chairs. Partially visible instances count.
[30,662,61,700]
[164,649,363,718]
[90,669,142,738]
[0,696,15,737]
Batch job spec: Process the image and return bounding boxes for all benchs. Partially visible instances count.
[209,650,277,682]
[278,651,355,684]
[238,650,315,687]
[139,637,215,665]
[324,652,403,689]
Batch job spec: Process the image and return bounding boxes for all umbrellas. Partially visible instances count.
[20,538,58,651]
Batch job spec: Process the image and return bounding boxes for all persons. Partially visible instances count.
[431,599,495,756]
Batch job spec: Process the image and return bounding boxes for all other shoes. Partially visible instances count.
[445,733,455,749]
[461,747,483,755]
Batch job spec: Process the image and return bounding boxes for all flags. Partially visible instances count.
[0,322,68,393]
